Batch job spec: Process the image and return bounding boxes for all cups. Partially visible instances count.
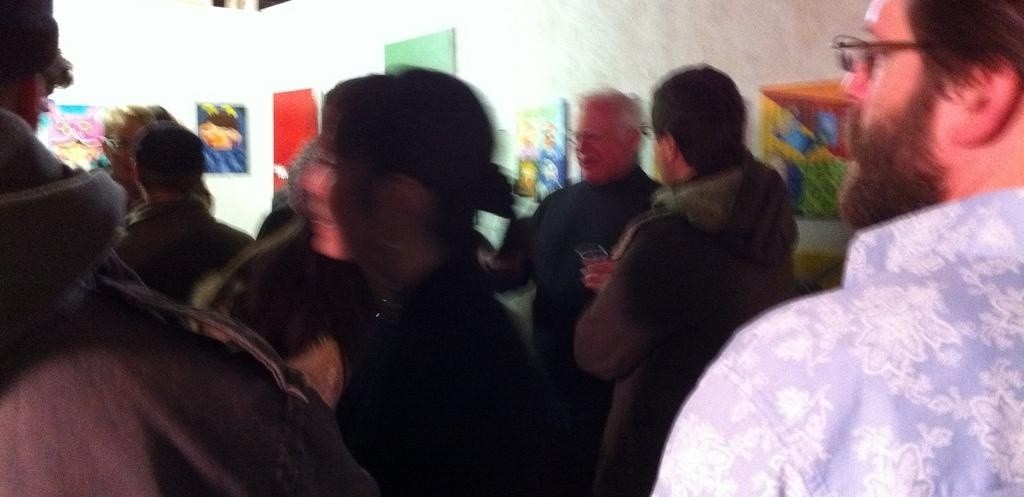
[581,249,609,276]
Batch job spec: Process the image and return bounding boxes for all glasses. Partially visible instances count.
[826,34,922,72]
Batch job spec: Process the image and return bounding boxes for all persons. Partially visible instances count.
[571,54,799,496]
[1,0,545,496]
[646,0,1024,496]
[486,85,665,448]
